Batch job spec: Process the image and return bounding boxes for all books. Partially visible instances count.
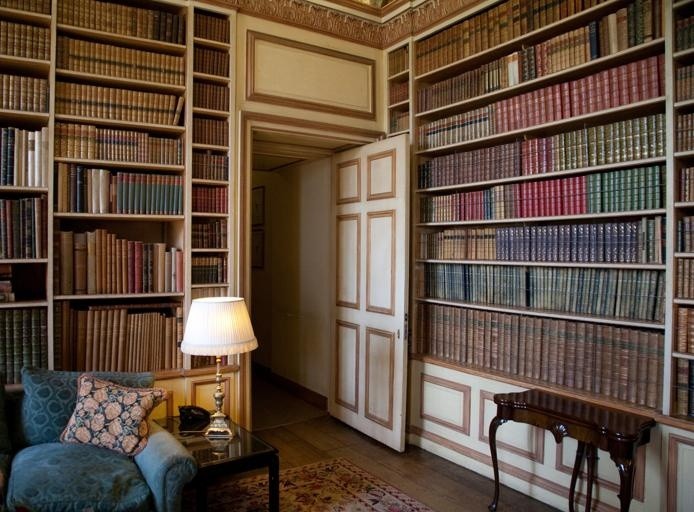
[56,82,185,126]
[57,37,185,86]
[58,0,185,45]
[55,124,183,165]
[387,44,410,133]
[53,229,183,295]
[54,162,183,215]
[54,301,183,372]
[674,17,694,418]
[191,13,230,299]
[0,1,51,388]
[413,0,665,414]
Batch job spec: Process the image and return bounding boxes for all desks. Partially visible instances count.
[151,411,281,512]
[487,389,655,511]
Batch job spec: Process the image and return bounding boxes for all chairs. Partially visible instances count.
[1,367,200,512]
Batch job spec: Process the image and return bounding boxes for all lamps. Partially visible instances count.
[179,296,259,438]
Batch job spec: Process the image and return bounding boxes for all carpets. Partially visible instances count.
[183,456,436,512]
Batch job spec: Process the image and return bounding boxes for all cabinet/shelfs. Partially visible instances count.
[385,0,694,434]
[0,1,239,393]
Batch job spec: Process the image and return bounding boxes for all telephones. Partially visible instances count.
[178,405,210,422]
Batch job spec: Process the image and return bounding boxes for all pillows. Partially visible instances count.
[60,373,168,457]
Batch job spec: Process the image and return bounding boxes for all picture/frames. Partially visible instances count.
[252,185,266,269]
